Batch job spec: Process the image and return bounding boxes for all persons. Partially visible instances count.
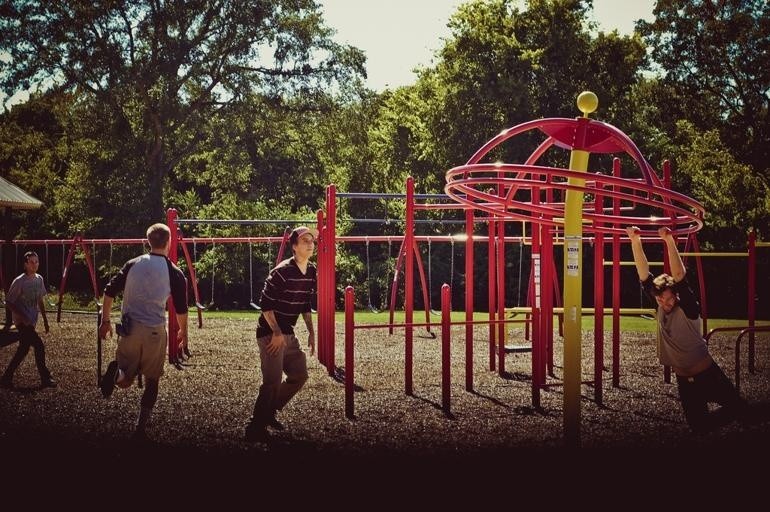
[246,225,320,444]
[626,225,745,436]
[98,223,188,444]
[0,251,58,387]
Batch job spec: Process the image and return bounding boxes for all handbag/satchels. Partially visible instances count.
[103,320,110,324]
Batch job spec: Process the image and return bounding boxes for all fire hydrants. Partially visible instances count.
[495,242,523,320]
[249,243,272,310]
[91,242,114,307]
[45,244,65,307]
[366,241,394,313]
[193,242,215,311]
[429,241,455,315]
[641,281,658,320]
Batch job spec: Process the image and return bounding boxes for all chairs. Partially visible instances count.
[100,361,119,398]
[41,377,57,388]
[0,376,16,391]
[244,415,284,443]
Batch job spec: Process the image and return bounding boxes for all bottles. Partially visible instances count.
[288,226,320,246]
